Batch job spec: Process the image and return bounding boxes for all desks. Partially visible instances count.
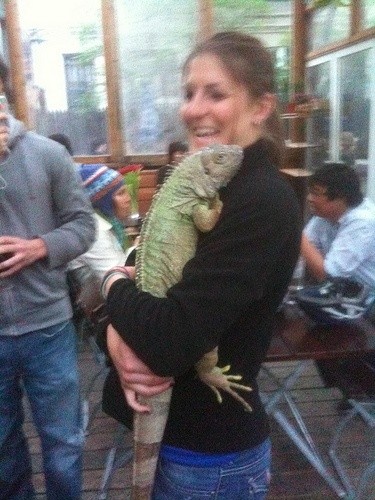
[257,278,375,500]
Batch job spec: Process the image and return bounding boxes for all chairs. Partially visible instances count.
[70,265,136,500]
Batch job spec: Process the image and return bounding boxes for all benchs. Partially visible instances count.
[134,169,161,218]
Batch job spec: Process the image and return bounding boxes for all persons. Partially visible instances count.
[45,132,189,350]
[272,162,375,335]
[0,55,98,500]
[88,32,302,500]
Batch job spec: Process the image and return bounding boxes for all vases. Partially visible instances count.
[128,214,141,227]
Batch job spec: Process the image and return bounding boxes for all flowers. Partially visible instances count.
[120,163,144,217]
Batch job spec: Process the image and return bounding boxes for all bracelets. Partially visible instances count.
[97,265,129,303]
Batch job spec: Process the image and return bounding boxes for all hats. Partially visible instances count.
[77,162,126,251]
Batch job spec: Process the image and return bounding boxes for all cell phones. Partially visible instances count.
[0,96,9,128]
